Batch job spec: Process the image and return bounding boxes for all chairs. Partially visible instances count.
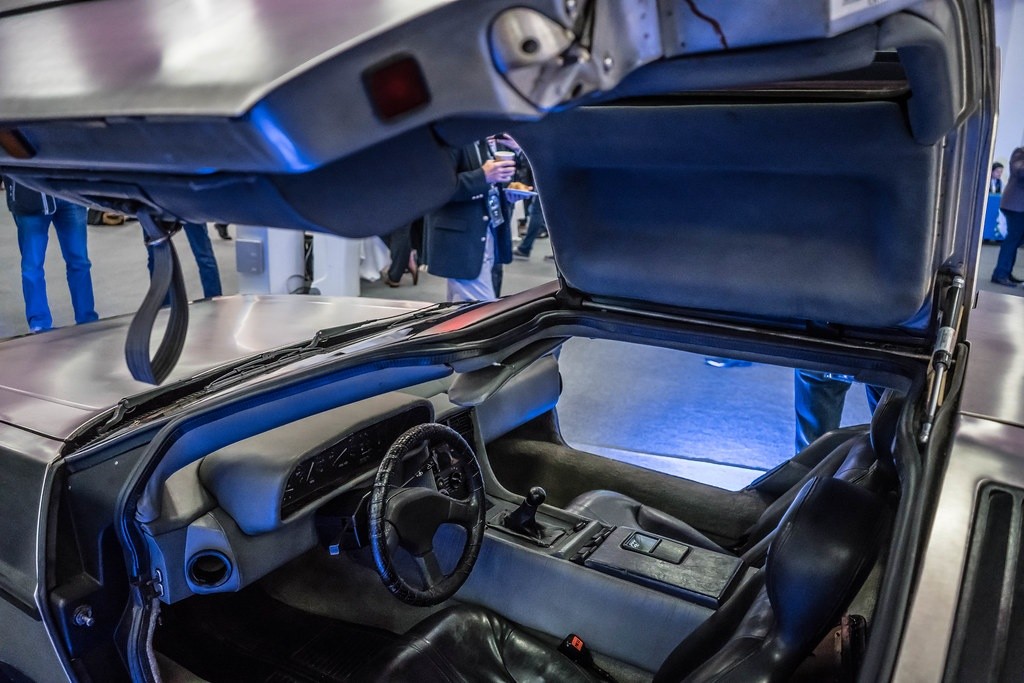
[364,476,893,683]
[561,386,907,570]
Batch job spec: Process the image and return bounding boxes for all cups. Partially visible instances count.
[494,151,515,162]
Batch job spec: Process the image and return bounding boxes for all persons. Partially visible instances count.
[3,176,99,333]
[266,228,361,298]
[214,223,232,240]
[796,368,887,456]
[385,131,544,304]
[990,147,1024,288]
[989,162,1004,194]
[143,221,222,305]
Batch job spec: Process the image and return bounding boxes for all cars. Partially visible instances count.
[0,0,1024,683]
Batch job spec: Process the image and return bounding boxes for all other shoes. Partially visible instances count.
[386,279,399,287]
[1010,275,1023,284]
[991,275,1017,287]
[512,247,529,261]
[408,249,420,285]
[213,223,231,240]
[545,256,555,262]
